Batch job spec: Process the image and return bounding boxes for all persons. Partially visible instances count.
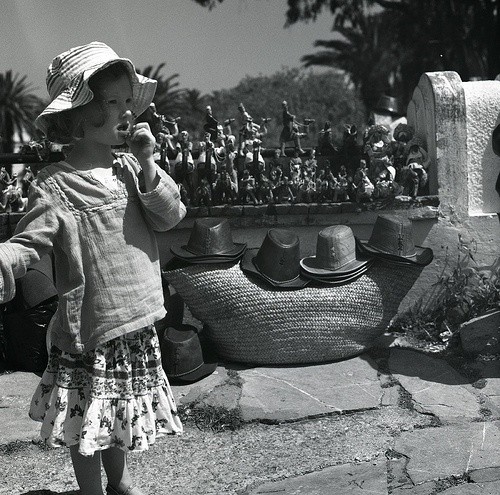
[1,97,432,204]
[0,40,189,495]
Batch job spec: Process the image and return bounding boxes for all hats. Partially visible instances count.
[242,228,311,287]
[35,41,158,144]
[300,225,373,283]
[354,214,434,268]
[170,216,247,263]
[373,94,406,117]
